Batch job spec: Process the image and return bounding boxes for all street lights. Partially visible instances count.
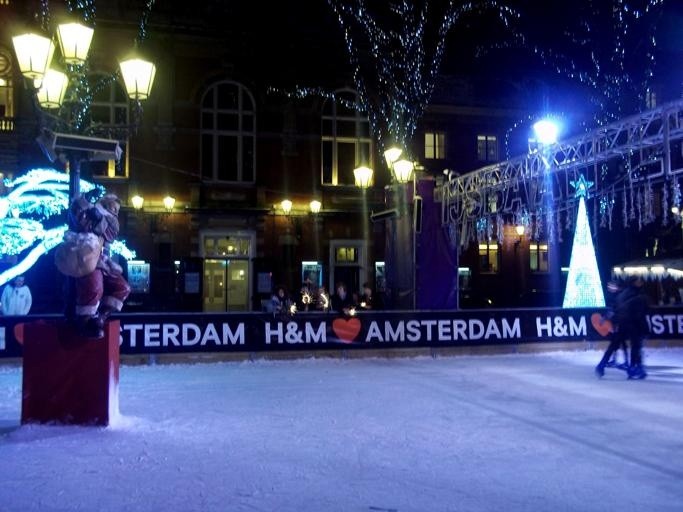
[1,5,159,430]
[354,131,414,314]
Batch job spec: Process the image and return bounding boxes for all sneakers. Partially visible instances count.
[596,360,647,379]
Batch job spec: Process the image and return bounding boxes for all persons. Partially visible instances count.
[266,278,379,314]
[601,272,683,308]
[594,275,646,378]
[606,280,630,370]
[71,189,131,338]
[1,276,33,337]
